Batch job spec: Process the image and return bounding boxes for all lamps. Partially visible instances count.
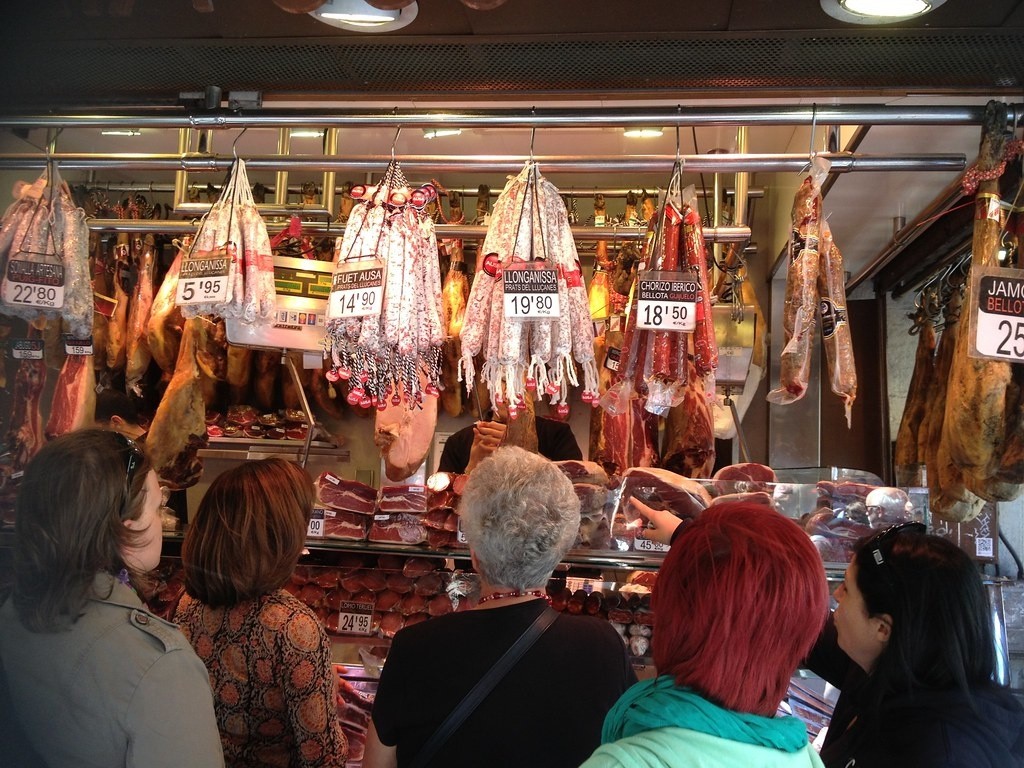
[820,0,947,25]
[308,0,419,33]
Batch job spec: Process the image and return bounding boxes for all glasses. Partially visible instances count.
[864,504,878,511]
[101,430,144,516]
[863,521,928,548]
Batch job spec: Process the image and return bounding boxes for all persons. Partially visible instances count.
[629,496,1024,768]
[363,445,639,767]
[94,389,149,449]
[0,428,225,768]
[576,503,829,768]
[438,377,583,474]
[176,456,356,768]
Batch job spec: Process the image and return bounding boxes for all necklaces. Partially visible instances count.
[475,590,554,606]
